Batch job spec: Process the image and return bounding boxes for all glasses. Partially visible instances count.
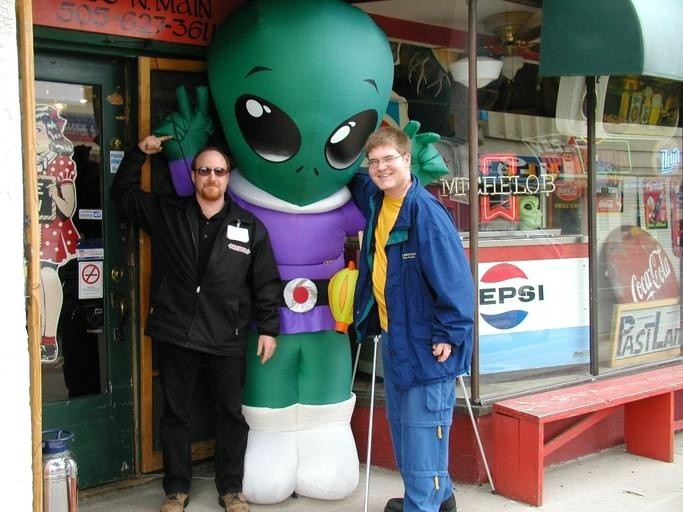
[193,167,231,176]
[368,154,406,169]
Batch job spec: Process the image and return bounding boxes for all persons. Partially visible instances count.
[348,126,475,512]
[109,134,281,512]
[56,144,104,398]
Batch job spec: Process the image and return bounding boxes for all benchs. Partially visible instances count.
[492,364,683,507]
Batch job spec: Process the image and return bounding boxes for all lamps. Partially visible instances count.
[497,46,525,85]
[448,54,503,91]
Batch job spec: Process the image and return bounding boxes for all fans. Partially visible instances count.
[431,11,543,56]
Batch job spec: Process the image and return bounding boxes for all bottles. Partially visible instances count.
[647,190,665,222]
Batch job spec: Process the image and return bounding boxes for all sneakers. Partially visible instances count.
[384,491,457,512]
[159,492,189,512]
[218,491,250,512]
